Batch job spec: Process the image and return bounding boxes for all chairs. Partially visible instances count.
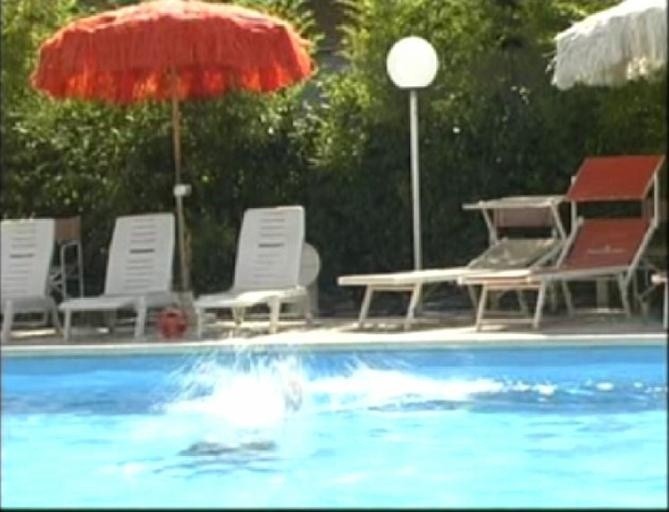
[336,261,561,333]
[455,194,576,333]
[57,211,189,344]
[192,204,314,338]
[0,218,63,344]
[551,150,667,322]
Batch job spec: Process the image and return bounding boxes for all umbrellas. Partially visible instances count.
[33,0,313,293]
[551,1,669,92]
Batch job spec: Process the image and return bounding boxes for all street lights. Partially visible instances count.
[386,36,439,320]
